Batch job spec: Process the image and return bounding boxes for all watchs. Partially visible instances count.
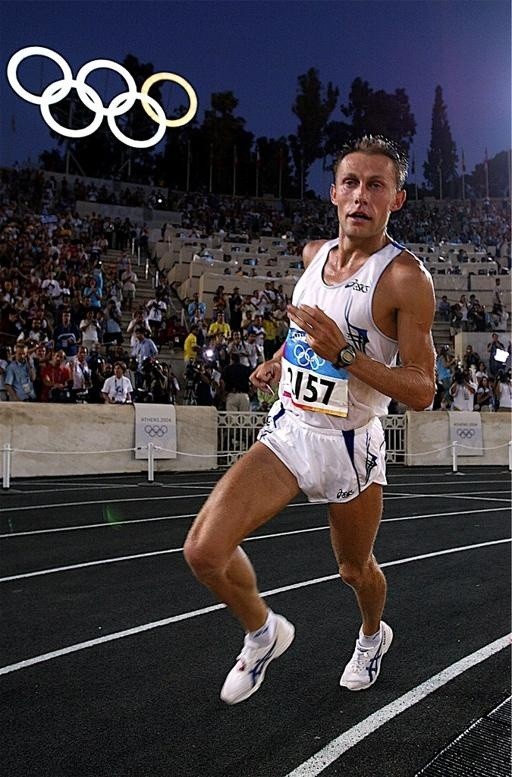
[330,343,356,370]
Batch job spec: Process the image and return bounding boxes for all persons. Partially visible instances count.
[183,138,435,704]
[0,169,510,411]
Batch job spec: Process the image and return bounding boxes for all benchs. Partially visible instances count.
[0,221,512,417]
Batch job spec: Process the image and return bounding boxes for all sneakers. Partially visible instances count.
[220,607,295,706]
[339,620,393,691]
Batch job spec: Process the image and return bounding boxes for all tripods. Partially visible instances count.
[183,380,198,405]
